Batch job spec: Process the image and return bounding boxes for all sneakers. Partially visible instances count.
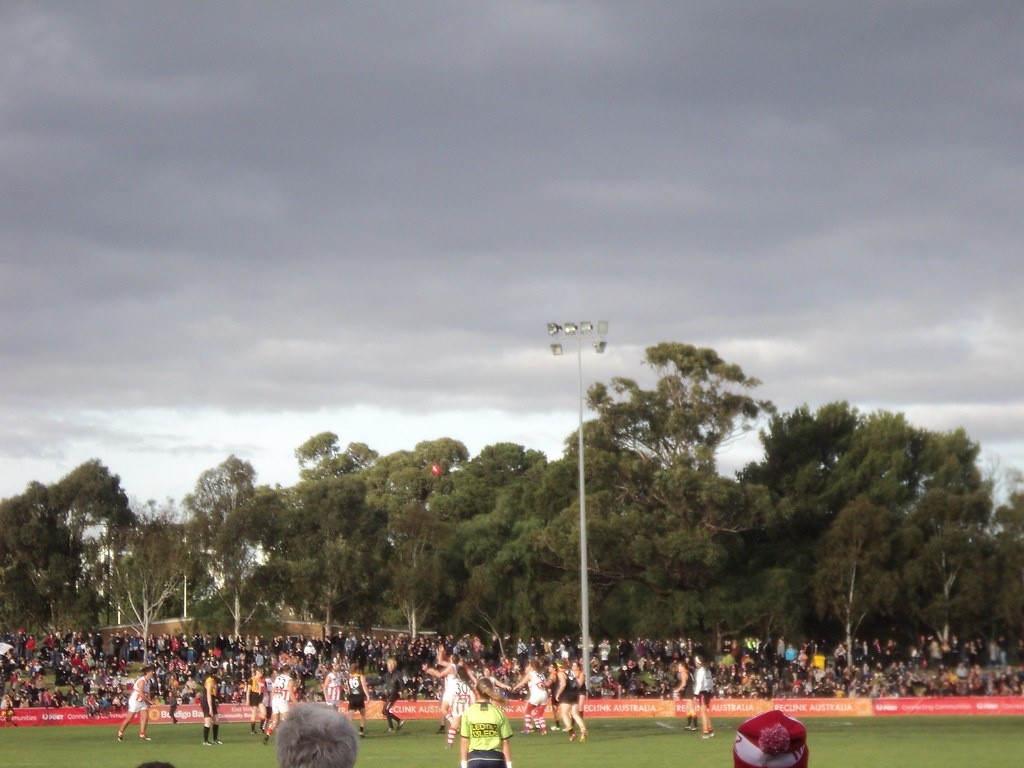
[172,719,177,724]
[519,725,588,743]
[202,740,222,745]
[358,732,365,737]
[117,733,152,743]
[684,725,715,739]
[383,720,405,732]
[251,728,269,745]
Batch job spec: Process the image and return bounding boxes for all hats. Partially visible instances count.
[732,710,810,768]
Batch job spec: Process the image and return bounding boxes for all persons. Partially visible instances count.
[366,659,405,733]
[168,692,179,725]
[732,709,811,767]
[346,663,370,739]
[0,630,1023,718]
[675,661,700,731]
[263,664,297,745]
[423,644,460,733]
[538,662,569,732]
[117,668,155,742]
[460,678,513,768]
[260,668,277,731]
[692,656,718,739]
[556,659,588,743]
[246,667,267,734]
[201,669,223,745]
[571,663,585,728]
[514,659,544,735]
[277,701,359,768]
[524,666,541,733]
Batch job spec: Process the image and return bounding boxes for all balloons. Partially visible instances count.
[431,465,441,476]
